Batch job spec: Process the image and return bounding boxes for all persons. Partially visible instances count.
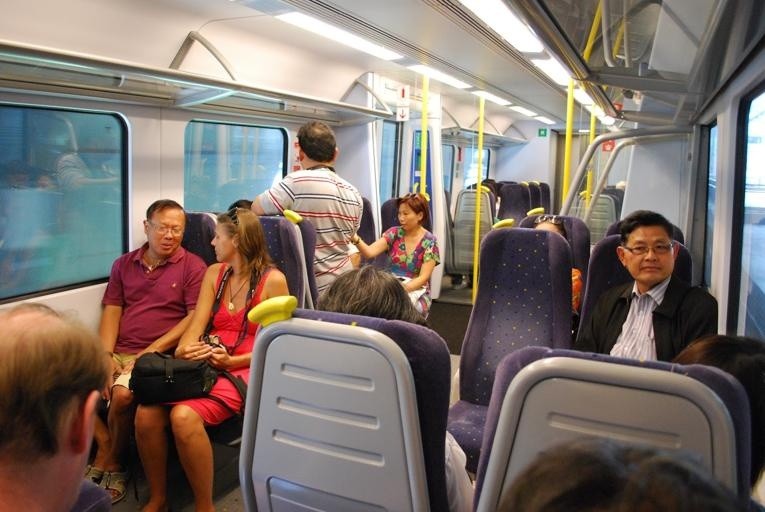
[0,303,108,512]
[251,121,364,297]
[350,192,441,318]
[317,264,474,512]
[82,200,208,505]
[574,210,718,363]
[0,124,120,288]
[497,442,734,512]
[532,215,584,316]
[231,199,254,210]
[134,206,290,512]
[672,335,765,488]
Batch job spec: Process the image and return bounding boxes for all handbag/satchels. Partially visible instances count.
[128,351,216,400]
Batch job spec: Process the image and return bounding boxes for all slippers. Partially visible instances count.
[83,463,128,504]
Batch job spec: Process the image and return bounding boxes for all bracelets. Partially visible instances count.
[353,238,360,246]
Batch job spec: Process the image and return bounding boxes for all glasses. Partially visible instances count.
[624,241,674,255]
[405,192,420,200]
[145,220,183,237]
[228,207,239,226]
[534,214,563,225]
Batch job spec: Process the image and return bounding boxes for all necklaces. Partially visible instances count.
[228,278,248,311]
